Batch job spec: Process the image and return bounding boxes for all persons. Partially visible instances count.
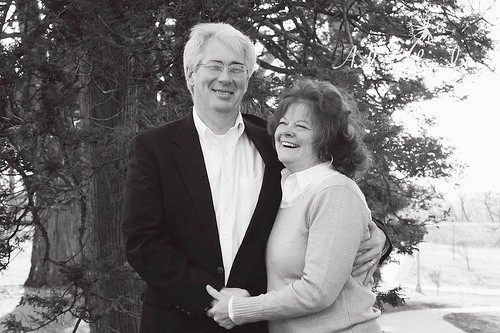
[206,77,385,333]
[121,22,393,333]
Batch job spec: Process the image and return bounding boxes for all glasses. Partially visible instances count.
[198,63,249,76]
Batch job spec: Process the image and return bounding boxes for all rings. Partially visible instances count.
[370,260,375,266]
